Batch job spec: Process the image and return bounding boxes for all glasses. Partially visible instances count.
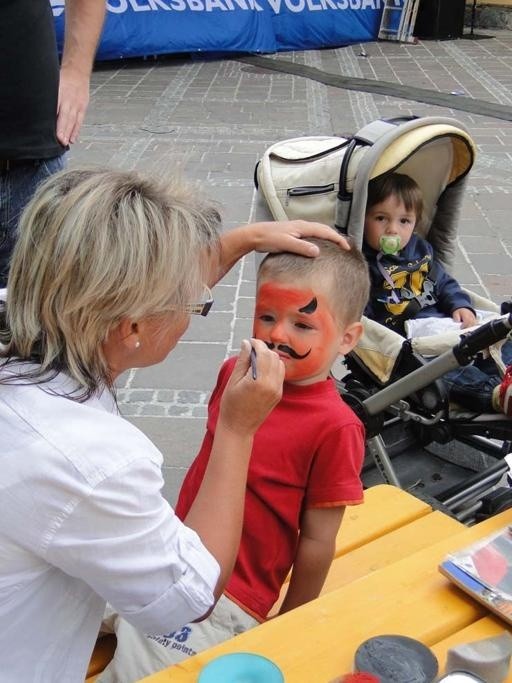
[156,283,214,316]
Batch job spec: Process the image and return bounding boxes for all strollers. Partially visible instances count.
[246,109,512,529]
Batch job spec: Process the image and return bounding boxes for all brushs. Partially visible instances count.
[245,345,259,382]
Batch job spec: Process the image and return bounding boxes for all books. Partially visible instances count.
[436,523,512,625]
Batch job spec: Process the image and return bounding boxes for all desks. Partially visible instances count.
[142,505,509,683]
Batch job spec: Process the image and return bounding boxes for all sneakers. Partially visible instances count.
[499,366,512,417]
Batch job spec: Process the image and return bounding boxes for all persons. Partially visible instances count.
[85,234,371,683]
[0,166,351,683]
[0,0,107,290]
[364,171,512,416]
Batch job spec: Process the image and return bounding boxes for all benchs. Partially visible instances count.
[87,482,468,683]
